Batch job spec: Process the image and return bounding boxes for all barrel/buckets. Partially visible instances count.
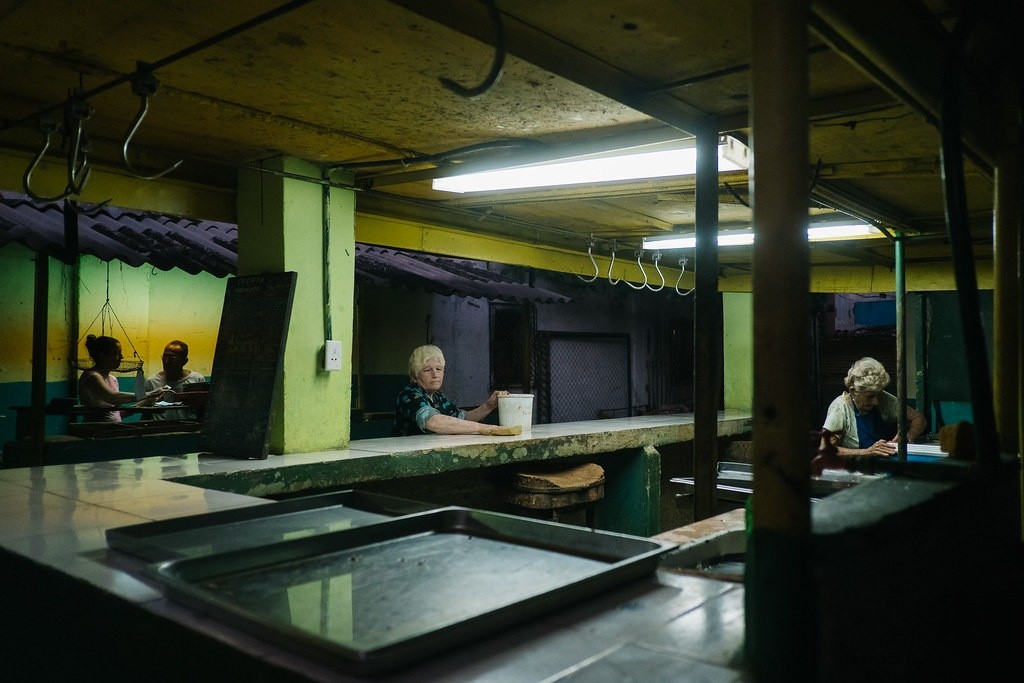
[497,394,534,436]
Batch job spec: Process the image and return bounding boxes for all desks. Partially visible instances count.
[52,399,189,421]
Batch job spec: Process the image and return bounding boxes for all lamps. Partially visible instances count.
[642,221,881,251]
[431,128,749,194]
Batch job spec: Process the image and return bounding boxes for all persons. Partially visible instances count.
[79,335,139,423]
[392,344,508,505]
[143,340,208,421]
[818,357,928,476]
